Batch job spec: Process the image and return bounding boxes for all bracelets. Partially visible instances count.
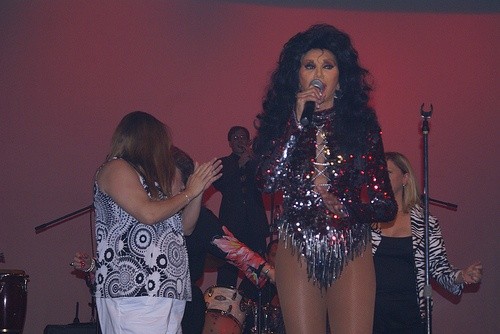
[183,191,191,204]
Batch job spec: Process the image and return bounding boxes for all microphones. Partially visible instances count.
[300,79,323,126]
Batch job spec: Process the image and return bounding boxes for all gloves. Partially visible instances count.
[212,226,265,270]
[225,253,267,289]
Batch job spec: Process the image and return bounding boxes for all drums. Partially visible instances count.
[202,285,249,334]
[0,268,29,334]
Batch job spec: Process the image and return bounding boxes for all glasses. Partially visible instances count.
[232,135,246,140]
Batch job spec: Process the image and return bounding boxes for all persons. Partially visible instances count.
[212,127,270,289]
[370,152,482,334]
[165,146,275,334]
[93,111,223,334]
[238,240,281,334]
[252,23,398,334]
